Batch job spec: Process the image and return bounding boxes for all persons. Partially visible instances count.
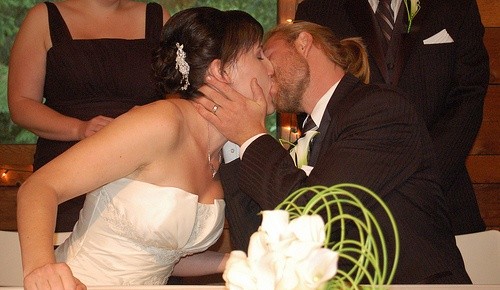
[294,0,490,235]
[17,6,275,290]
[190,21,473,285]
[8,0,181,233]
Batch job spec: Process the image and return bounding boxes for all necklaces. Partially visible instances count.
[208,140,222,178]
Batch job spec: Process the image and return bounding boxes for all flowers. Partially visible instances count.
[404,0,422,34]
[279,131,321,176]
[223,209,339,290]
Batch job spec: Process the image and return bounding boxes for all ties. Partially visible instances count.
[289,116,315,164]
[375,0,394,47]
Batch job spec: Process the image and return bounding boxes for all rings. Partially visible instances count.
[211,105,220,114]
